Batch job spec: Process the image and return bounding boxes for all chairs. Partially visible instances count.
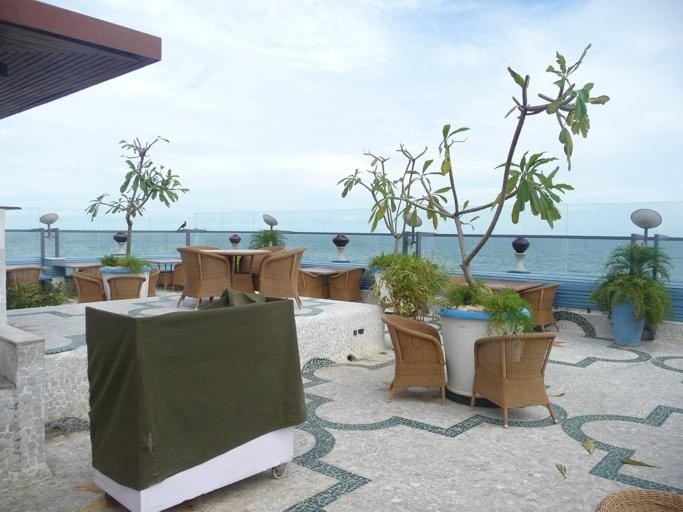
[7,266,43,301]
[519,284,560,333]
[259,247,306,306]
[176,247,231,309]
[471,332,557,428]
[144,260,186,291]
[72,261,162,301]
[240,246,286,274]
[381,314,447,407]
[328,267,364,303]
[297,268,325,298]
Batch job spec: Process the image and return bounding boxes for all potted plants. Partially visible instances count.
[589,243,673,346]
[369,42,610,407]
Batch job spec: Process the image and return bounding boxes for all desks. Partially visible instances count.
[302,267,342,295]
[483,279,545,294]
[203,250,272,292]
